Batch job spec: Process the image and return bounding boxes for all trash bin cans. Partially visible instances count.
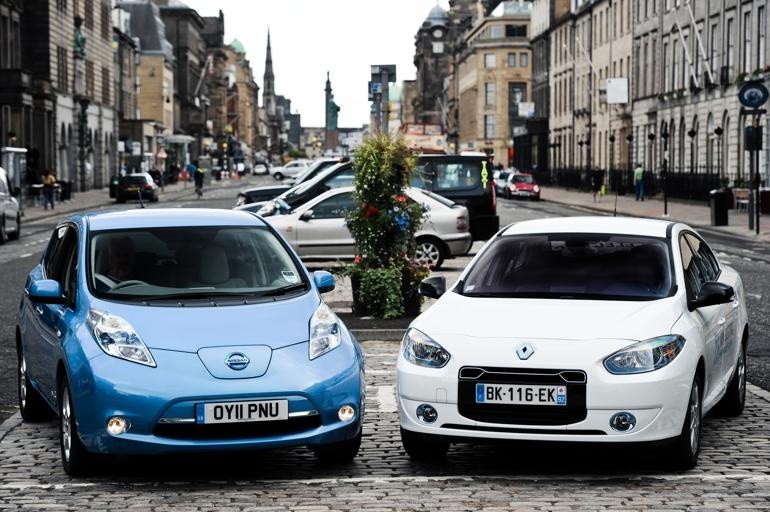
[60,180,71,200]
[709,189,728,225]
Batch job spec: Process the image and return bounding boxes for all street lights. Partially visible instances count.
[660,119,670,217]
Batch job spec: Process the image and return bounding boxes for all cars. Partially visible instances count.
[109,173,162,203]
[392,210,750,475]
[1,167,25,243]
[13,213,365,482]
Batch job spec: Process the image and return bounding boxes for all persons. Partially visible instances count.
[40,171,56,209]
[633,164,645,201]
[194,168,207,193]
[591,166,603,202]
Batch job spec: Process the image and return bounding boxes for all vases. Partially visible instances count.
[348,270,424,317]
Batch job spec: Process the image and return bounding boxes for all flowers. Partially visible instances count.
[324,124,436,319]
